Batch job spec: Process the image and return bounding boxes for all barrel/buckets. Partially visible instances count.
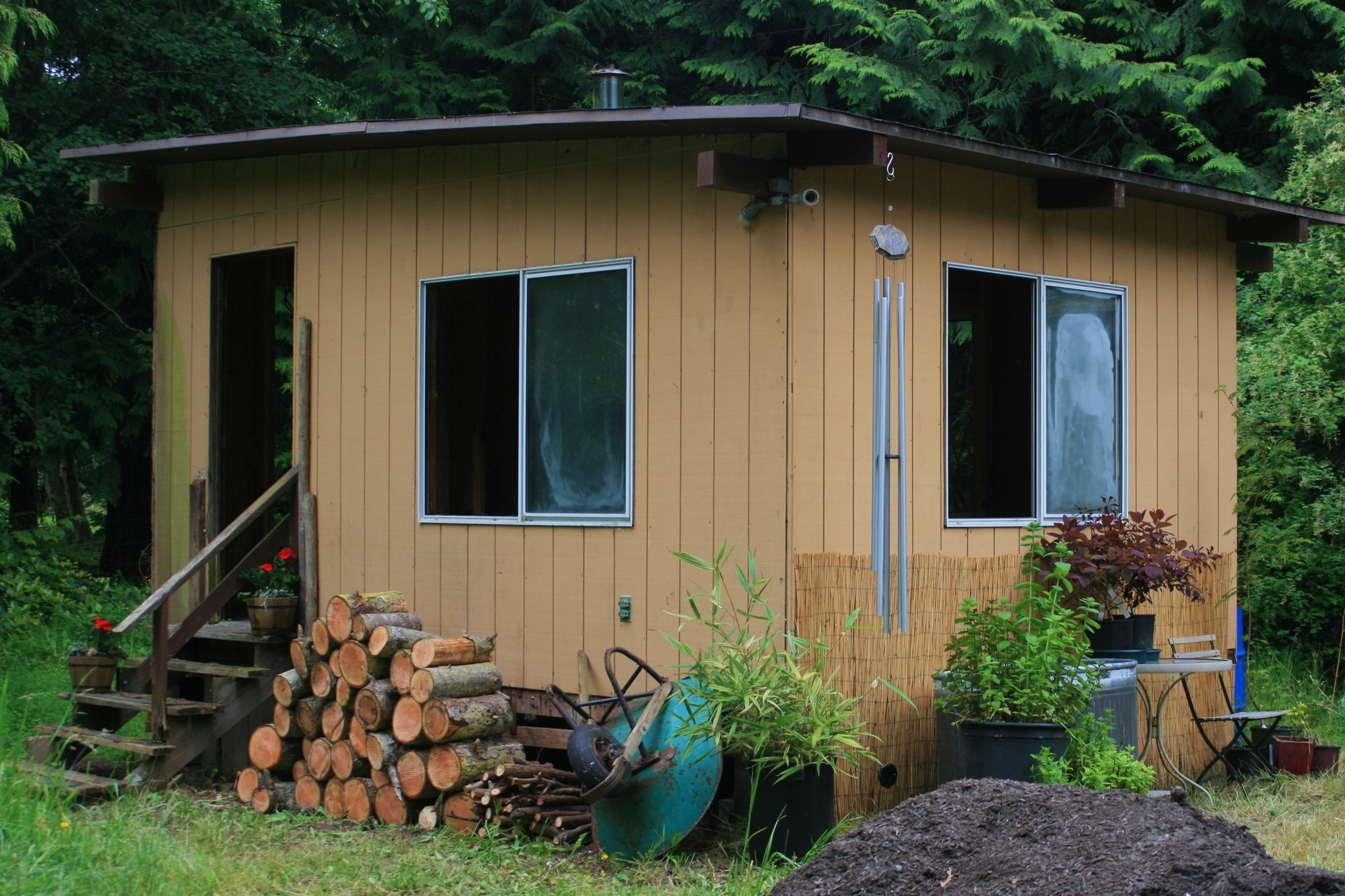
[1233,599,1246,747]
[1223,746,1267,785]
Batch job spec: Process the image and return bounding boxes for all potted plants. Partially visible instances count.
[1272,701,1314,776]
[932,509,1224,795]
[1214,383,1302,766]
[653,526,922,870]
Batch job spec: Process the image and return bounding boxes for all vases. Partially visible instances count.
[1313,744,1342,779]
[67,656,118,693]
[1223,744,1266,786]
[244,597,299,636]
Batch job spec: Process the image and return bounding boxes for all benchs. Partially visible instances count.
[1167,634,1295,800]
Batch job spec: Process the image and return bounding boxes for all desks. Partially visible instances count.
[1124,656,1232,805]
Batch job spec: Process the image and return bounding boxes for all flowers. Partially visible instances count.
[69,615,129,660]
[234,547,299,602]
